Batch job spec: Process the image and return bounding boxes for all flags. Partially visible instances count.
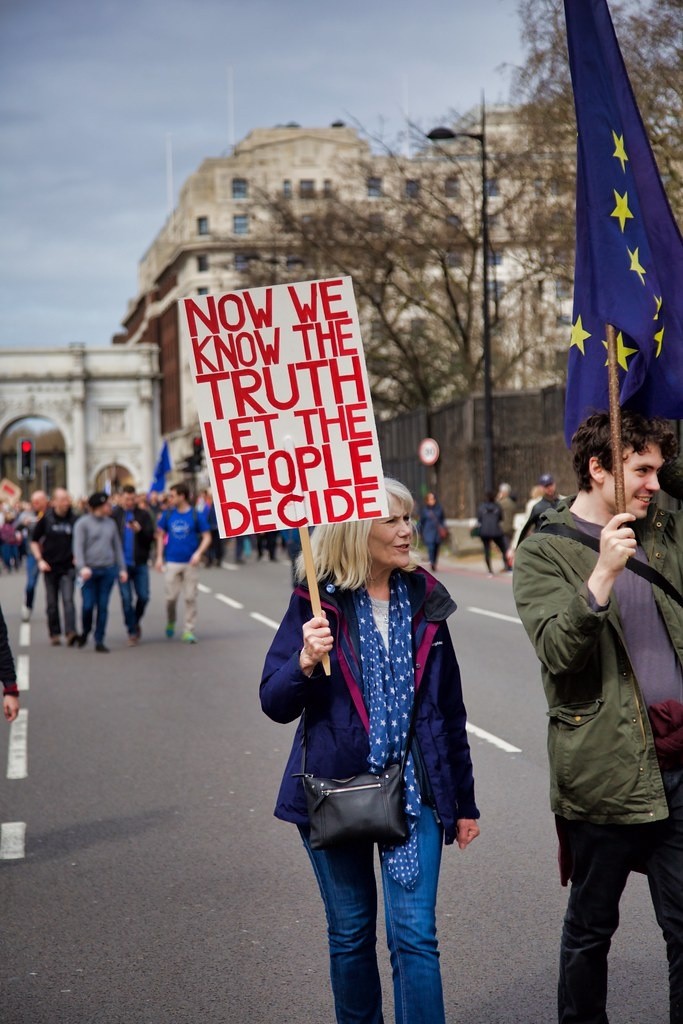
[562,0,683,450]
[147,442,171,492]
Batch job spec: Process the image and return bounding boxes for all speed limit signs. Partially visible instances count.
[420,439,438,465]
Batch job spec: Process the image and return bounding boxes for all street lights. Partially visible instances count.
[424,91,495,496]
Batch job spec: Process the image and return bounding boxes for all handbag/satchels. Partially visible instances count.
[305,765,410,849]
[439,528,450,538]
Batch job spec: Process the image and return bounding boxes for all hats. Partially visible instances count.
[540,474,554,486]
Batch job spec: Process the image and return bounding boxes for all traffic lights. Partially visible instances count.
[193,437,205,472]
[16,437,36,480]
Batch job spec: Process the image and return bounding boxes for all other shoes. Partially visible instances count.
[96,643,110,653]
[79,633,87,648]
[22,605,32,621]
[181,630,198,642]
[128,637,136,647]
[66,634,78,647]
[166,621,175,637]
[486,573,494,579]
[499,569,513,576]
[216,554,278,568]
[52,641,62,647]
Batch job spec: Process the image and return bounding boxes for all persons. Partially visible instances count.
[0,604,21,724]
[416,492,444,571]
[516,473,566,551]
[476,482,517,573]
[0,485,311,653]
[259,478,481,1024]
[513,407,683,1024]
